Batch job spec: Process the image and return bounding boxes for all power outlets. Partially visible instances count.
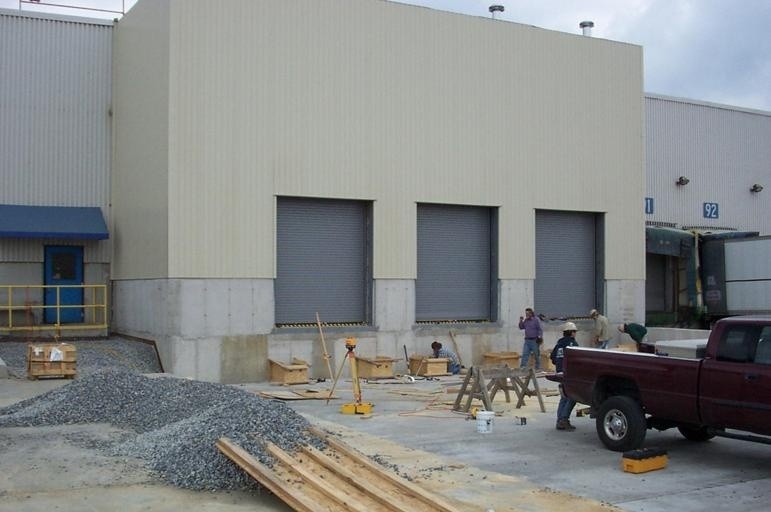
[541,314,767,452]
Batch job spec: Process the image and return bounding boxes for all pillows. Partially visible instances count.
[590,309,596,315]
[563,322,577,333]
[618,323,626,332]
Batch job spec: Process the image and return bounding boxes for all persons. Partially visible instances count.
[518,307,544,373]
[589,308,611,351]
[618,321,650,344]
[432,340,464,374]
[549,320,581,431]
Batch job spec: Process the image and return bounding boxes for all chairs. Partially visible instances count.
[556,422,576,429]
[560,417,573,431]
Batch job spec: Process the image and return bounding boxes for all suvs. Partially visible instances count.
[475,411,496,433]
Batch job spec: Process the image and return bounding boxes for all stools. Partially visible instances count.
[525,337,537,340]
